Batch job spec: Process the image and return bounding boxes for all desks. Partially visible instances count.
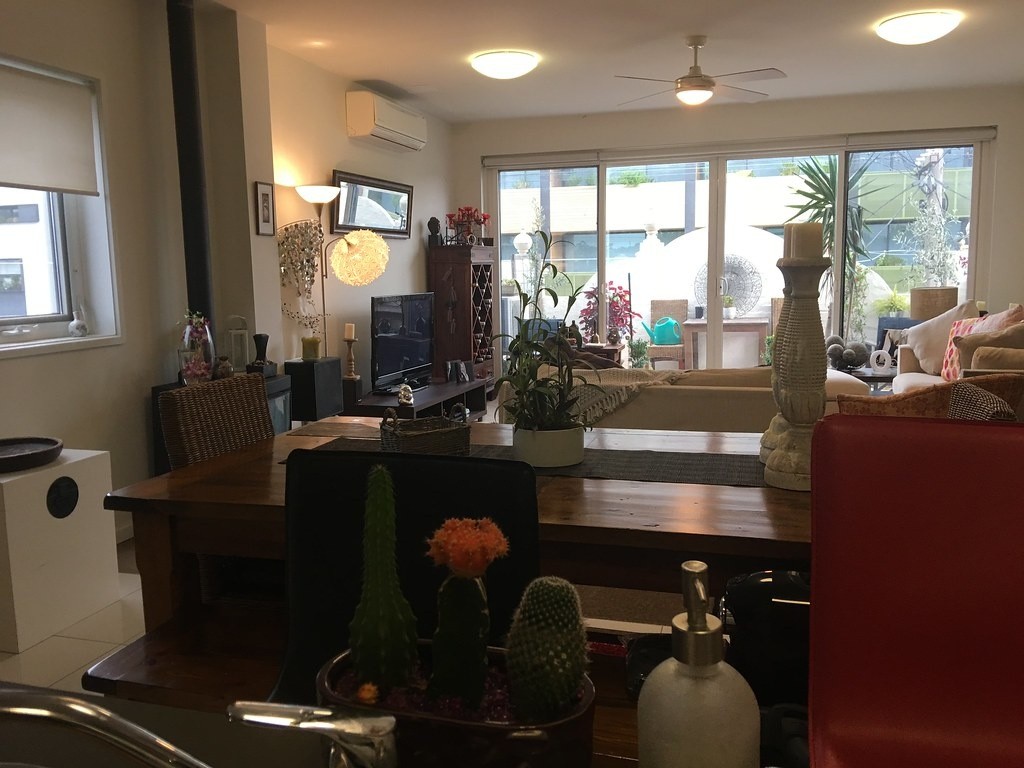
[682,318,770,370]
[284,356,345,422]
[876,316,924,345]
[0,448,121,654]
[570,343,625,368]
[150,374,291,477]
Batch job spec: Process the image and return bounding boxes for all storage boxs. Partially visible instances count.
[224,314,250,373]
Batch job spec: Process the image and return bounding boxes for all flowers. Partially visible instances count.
[342,465,592,723]
[578,280,643,344]
[176,307,212,381]
[415,302,425,317]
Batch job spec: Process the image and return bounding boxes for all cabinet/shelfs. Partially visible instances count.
[81,416,810,768]
[353,379,488,423]
[425,244,496,401]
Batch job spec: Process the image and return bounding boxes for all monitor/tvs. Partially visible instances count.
[371,291,435,395]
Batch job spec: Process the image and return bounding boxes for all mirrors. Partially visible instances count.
[330,169,414,239]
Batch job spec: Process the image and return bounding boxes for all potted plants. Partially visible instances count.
[722,296,736,319]
[491,228,605,468]
[865,284,910,318]
[501,279,517,296]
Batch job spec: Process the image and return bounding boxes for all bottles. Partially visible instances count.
[68,311,88,337]
[569,320,578,338]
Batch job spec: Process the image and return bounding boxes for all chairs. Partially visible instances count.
[646,300,688,369]
[945,382,1018,421]
[810,414,1024,768]
[771,297,785,335]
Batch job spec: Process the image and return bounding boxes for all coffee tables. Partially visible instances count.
[830,367,897,396]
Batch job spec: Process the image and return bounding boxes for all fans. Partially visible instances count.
[613,35,788,107]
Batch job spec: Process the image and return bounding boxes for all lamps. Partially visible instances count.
[910,286,958,322]
[324,229,390,287]
[295,184,341,355]
[676,89,714,106]
[471,51,538,79]
[876,9,963,45]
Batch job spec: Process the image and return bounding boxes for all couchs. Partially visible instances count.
[354,195,396,228]
[497,300,1024,433]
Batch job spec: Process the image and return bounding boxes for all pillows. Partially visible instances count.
[970,345,1024,370]
[971,305,1024,334]
[941,314,990,382]
[902,300,980,375]
[953,319,1024,370]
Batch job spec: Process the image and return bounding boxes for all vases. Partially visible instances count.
[763,257,833,492]
[314,638,595,768]
[177,323,215,383]
[759,258,793,465]
[416,317,425,333]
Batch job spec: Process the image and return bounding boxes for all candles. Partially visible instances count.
[344,323,355,339]
[782,223,799,259]
[301,335,321,358]
[791,221,824,258]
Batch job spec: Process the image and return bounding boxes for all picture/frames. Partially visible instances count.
[254,180,275,236]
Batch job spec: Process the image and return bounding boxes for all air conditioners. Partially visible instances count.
[346,90,428,153]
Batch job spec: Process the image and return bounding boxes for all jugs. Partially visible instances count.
[642,317,682,345]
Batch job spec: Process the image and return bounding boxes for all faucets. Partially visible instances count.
[0,682,399,768]
